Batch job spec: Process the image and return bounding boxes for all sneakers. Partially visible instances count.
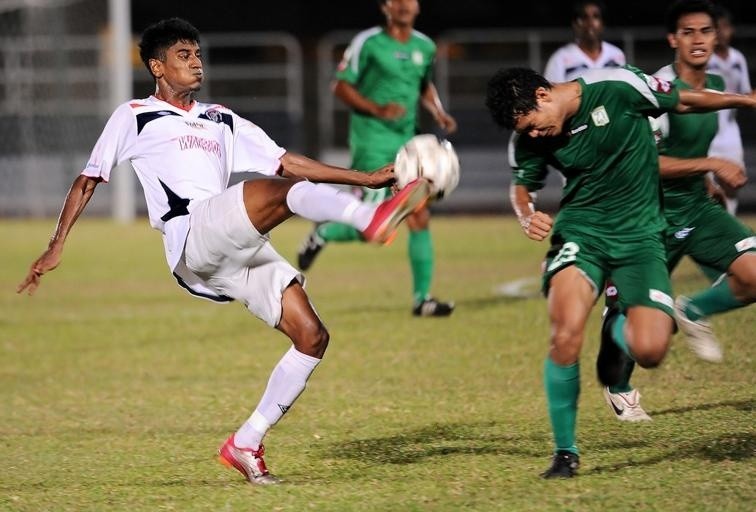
[675,294,723,362]
[299,234,324,270]
[597,309,654,421]
[541,452,579,479]
[218,432,285,486]
[412,298,454,317]
[363,178,429,246]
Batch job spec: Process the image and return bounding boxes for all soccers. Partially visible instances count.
[395,133,460,200]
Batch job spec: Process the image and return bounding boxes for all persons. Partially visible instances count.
[293,0,456,318]
[701,3,755,218]
[541,0,629,83]
[484,63,756,480]
[595,1,756,425]
[15,15,433,488]
